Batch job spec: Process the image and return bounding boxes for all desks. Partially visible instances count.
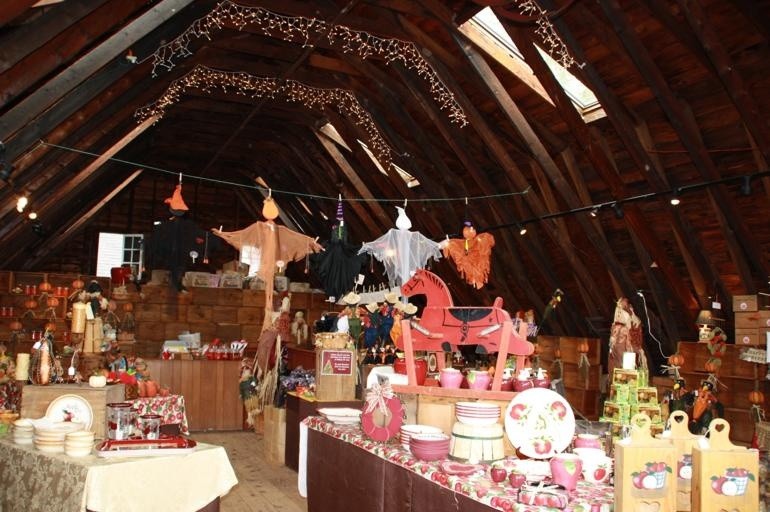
[144,359,243,431]
[1,431,237,512]
[297,417,770,512]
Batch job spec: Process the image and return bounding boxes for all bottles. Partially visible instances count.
[438,368,464,388]
[549,453,583,492]
[575,434,601,447]
[466,369,491,391]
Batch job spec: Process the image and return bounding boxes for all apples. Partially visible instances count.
[129,396,187,432]
[309,358,747,512]
[463,227,476,238]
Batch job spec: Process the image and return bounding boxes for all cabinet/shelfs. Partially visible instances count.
[0,271,110,359]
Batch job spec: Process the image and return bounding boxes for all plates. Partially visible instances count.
[504,386,577,461]
[399,400,501,463]
[316,406,363,426]
[12,394,95,459]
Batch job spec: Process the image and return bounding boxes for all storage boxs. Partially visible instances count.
[132,286,266,359]
[732,294,770,346]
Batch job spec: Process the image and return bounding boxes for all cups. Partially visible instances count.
[572,447,613,484]
[140,415,163,440]
[105,403,138,439]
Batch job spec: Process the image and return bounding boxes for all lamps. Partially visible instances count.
[29,208,37,219]
[610,202,623,221]
[695,310,714,343]
[669,186,681,207]
[740,176,753,196]
[513,221,527,236]
[590,206,600,217]
[12,187,27,215]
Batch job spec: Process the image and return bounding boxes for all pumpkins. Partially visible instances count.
[73,280,84,289]
[39,283,51,291]
[25,300,37,308]
[534,343,544,354]
[123,303,132,312]
[577,343,588,352]
[748,391,764,404]
[44,323,56,331]
[10,321,22,329]
[47,298,58,306]
